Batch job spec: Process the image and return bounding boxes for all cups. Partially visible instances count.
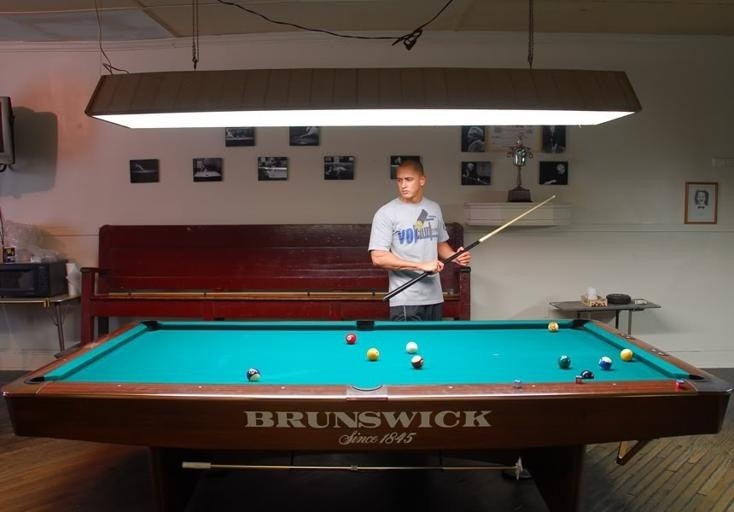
[588,288,597,299]
[65,261,78,298]
[2,246,14,264]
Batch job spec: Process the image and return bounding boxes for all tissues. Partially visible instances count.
[580,287,609,308]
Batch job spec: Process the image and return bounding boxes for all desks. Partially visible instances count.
[546,298,661,339]
[0,290,77,358]
[0,317,732,510]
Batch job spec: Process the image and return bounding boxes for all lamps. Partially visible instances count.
[79,0,644,133]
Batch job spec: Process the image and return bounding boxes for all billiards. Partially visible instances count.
[599,357,612,368]
[549,322,558,332]
[620,348,633,360]
[412,356,423,368]
[367,348,379,361]
[246,368,260,381]
[406,342,418,354]
[346,333,356,344]
[559,355,570,369]
[580,370,592,379]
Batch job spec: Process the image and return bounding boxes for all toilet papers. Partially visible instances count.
[65,261,82,298]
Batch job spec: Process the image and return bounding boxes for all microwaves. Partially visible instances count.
[0,258,69,297]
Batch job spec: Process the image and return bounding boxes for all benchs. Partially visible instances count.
[78,218,470,360]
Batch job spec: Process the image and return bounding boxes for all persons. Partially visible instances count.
[199,163,209,177]
[463,163,489,182]
[695,191,707,210]
[364,158,471,322]
[542,125,565,152]
[543,164,566,184]
[466,128,483,150]
[299,126,319,139]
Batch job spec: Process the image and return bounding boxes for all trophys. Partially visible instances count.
[504,133,534,203]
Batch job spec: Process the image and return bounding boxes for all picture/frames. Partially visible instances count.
[683,180,718,225]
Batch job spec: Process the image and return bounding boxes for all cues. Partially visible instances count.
[182,458,524,480]
[382,193,557,302]
